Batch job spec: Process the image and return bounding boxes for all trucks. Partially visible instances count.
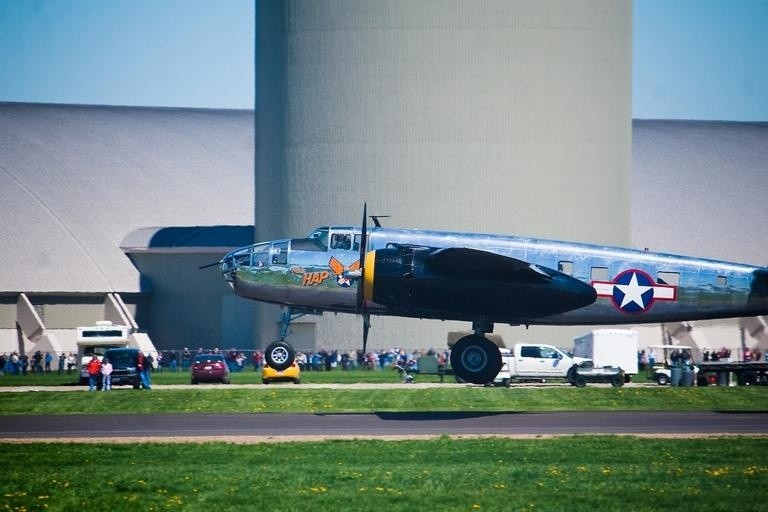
[574,330,639,381]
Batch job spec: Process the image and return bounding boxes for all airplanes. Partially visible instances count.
[198,205,768,384]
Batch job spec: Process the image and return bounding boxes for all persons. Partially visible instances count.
[639,349,649,370]
[156,350,164,371]
[670,350,679,365]
[677,349,690,364]
[182,346,191,372]
[66,351,77,373]
[86,354,101,393]
[168,349,178,372]
[146,353,153,371]
[222,347,268,373]
[208,347,222,354]
[294,346,452,383]
[134,350,151,390]
[702,346,768,362]
[331,233,350,249]
[45,351,53,373]
[98,354,114,391]
[647,347,657,365]
[198,347,203,355]
[0,350,44,377]
[58,352,66,375]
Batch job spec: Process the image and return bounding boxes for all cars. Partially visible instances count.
[192,353,230,384]
[262,360,301,384]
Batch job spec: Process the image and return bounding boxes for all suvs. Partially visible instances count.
[102,346,142,389]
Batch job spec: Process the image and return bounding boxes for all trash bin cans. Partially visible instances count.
[717,371,729,386]
[671,365,694,387]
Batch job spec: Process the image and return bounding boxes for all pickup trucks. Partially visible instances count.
[499,342,592,382]
